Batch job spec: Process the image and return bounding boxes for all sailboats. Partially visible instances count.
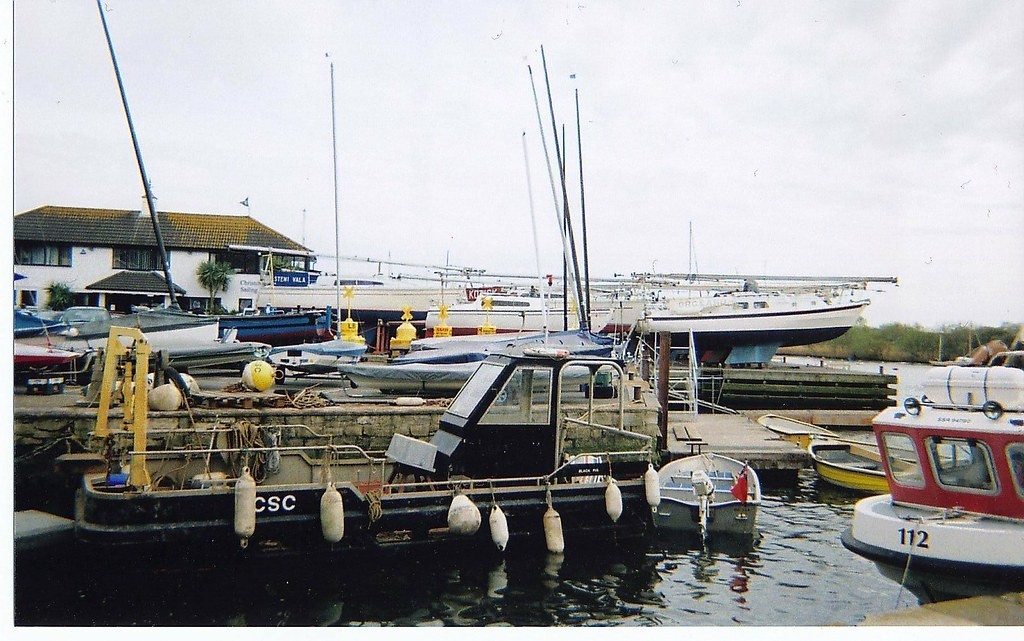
[13,0,618,402]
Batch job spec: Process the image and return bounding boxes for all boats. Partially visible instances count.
[758,411,838,447]
[648,452,762,541]
[244,249,899,366]
[808,436,957,494]
[68,349,663,560]
[838,349,1024,584]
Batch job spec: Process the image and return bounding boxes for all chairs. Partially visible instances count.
[218,325,237,344]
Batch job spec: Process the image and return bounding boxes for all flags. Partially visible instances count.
[729,464,748,504]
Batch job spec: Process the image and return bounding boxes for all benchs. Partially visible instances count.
[816,450,876,468]
